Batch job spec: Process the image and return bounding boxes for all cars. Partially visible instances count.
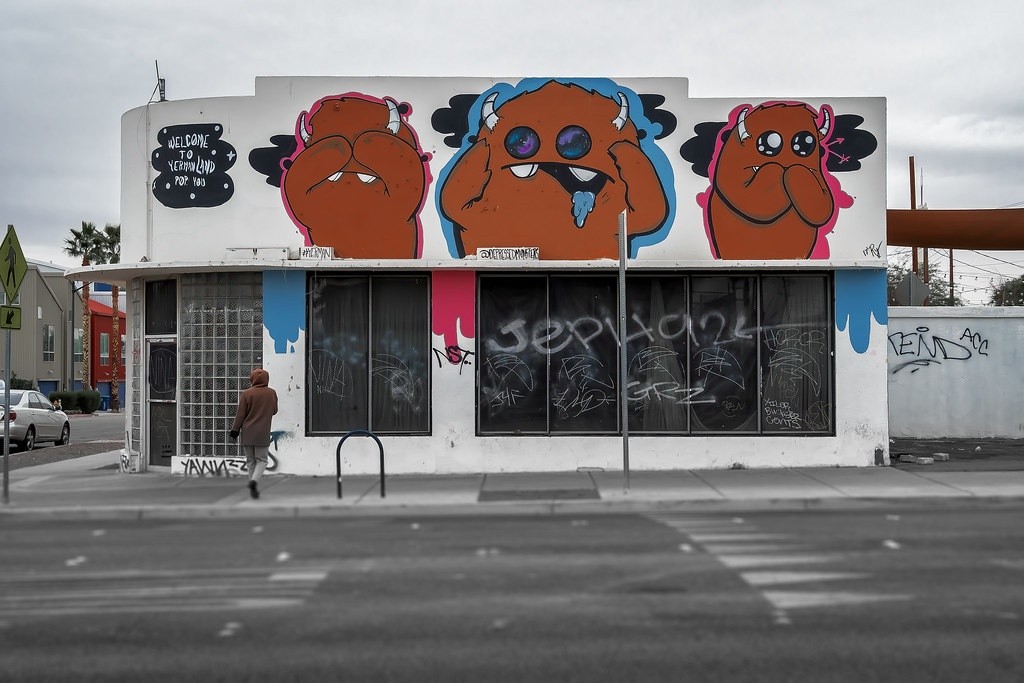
[0,389,72,451]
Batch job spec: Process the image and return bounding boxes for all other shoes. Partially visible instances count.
[247,480,260,499]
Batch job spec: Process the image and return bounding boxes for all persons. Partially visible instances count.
[231,370,278,498]
